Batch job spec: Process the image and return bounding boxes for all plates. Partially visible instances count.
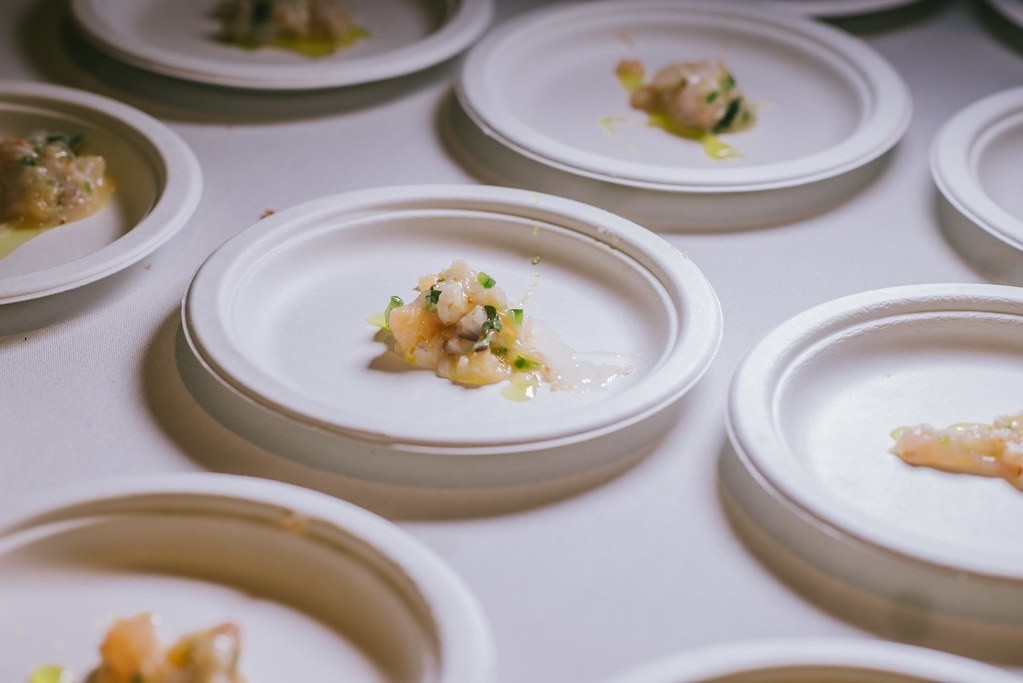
[927,84,1023,256]
[1,78,204,306]
[2,472,497,683]
[720,280,1023,584]
[451,0,914,193]
[179,182,725,458]
[602,636,1022,683]
[70,0,499,92]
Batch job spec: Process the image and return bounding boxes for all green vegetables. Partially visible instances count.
[704,71,749,134]
[384,272,529,370]
[21,132,84,167]
